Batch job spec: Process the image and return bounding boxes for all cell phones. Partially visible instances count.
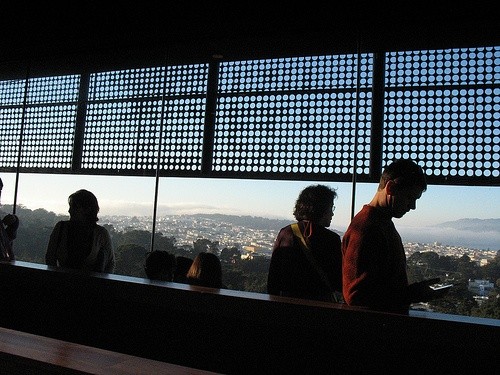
[430,283,453,290]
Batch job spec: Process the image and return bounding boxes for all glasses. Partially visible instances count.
[328,205,335,211]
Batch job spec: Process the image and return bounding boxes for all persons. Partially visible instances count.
[46,189,116,276]
[174,256,194,283]
[267,185,344,305]
[184,252,227,289]
[0,177,19,259]
[144,250,175,281]
[340,158,450,311]
